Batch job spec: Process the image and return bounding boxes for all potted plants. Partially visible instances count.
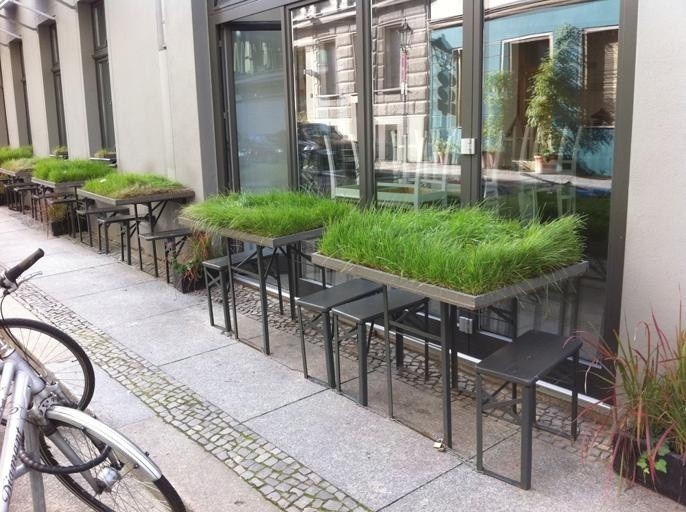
[564,292,685,509]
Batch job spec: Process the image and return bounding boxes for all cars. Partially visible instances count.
[295,121,360,187]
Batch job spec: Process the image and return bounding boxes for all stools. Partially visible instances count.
[0,179,93,240]
[297,278,431,407]
[139,228,200,284]
[76,206,156,266]
[203,247,283,332]
[474,330,583,490]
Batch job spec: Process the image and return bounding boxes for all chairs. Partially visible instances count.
[323,126,587,224]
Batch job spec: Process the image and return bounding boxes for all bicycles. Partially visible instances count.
[0,248,187,512]
[0,318,94,428]
